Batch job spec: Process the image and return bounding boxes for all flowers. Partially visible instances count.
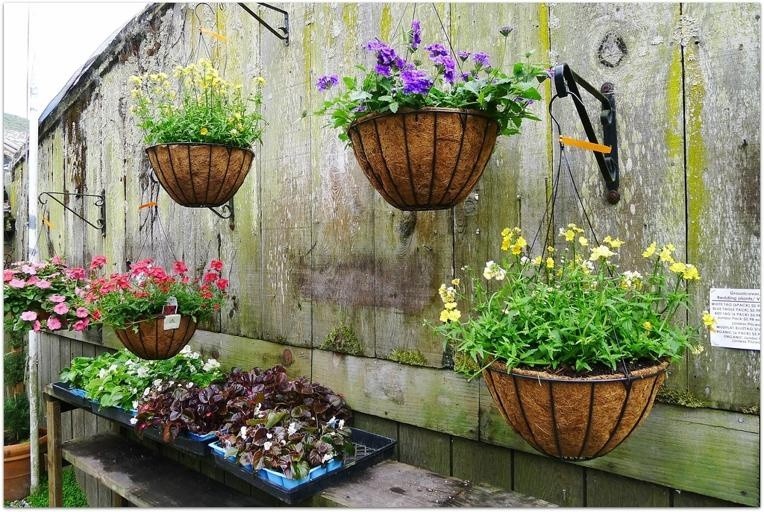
[4,254,104,333]
[59,346,221,426]
[427,222,716,375]
[129,367,351,442]
[304,22,554,137]
[128,61,267,150]
[217,404,354,477]
[90,252,229,328]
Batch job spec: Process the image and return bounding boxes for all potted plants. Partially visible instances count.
[4,322,47,502]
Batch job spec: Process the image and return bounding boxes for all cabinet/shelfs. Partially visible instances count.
[45,373,561,508]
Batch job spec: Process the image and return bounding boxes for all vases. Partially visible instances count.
[131,404,228,457]
[52,383,140,424]
[210,437,343,504]
[112,316,198,358]
[31,308,66,331]
[347,107,498,210]
[145,145,255,207]
[481,358,670,462]
